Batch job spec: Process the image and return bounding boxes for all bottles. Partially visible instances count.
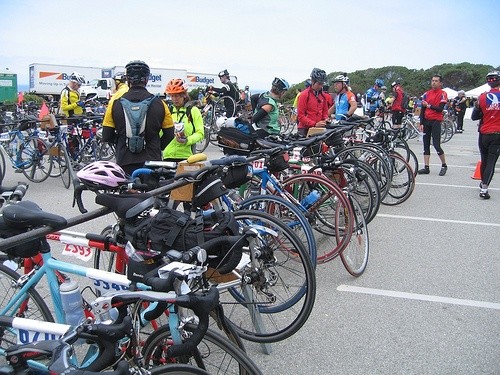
[60,277,86,327]
[298,189,322,213]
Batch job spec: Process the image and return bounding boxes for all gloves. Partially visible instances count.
[76,99,84,106]
[341,114,350,120]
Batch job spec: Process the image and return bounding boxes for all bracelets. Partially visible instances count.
[426,104,428,107]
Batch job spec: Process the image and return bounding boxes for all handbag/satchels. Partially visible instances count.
[300,137,324,157]
[223,163,254,189]
[145,206,239,270]
[323,128,344,146]
[267,150,290,173]
[191,165,228,208]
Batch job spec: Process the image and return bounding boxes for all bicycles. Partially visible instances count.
[293,95,462,145]
[1,98,294,189]
[1,197,269,375]
[78,149,319,341]
[245,140,419,274]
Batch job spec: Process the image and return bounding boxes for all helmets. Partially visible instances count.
[165,78,187,94]
[374,78,401,90]
[70,72,88,84]
[125,60,150,70]
[112,72,126,80]
[77,160,131,188]
[272,77,290,91]
[310,67,329,83]
[332,74,349,83]
[486,71,500,86]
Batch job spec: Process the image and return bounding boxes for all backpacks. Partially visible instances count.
[118,94,159,153]
[233,114,255,136]
[169,104,196,154]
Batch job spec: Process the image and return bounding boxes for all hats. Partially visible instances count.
[218,70,229,76]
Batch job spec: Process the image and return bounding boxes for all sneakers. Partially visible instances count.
[439,164,448,176]
[479,182,491,198]
[418,166,430,174]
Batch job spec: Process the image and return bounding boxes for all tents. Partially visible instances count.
[442,87,459,99]
[465,83,492,98]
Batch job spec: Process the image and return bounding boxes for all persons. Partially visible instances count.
[249,76,290,136]
[361,78,423,128]
[472,70,500,199]
[101,61,176,177]
[61,73,86,171]
[417,75,447,176]
[326,74,357,120]
[112,72,127,91]
[198,69,251,121]
[161,79,204,163]
[444,89,477,133]
[292,68,334,154]
